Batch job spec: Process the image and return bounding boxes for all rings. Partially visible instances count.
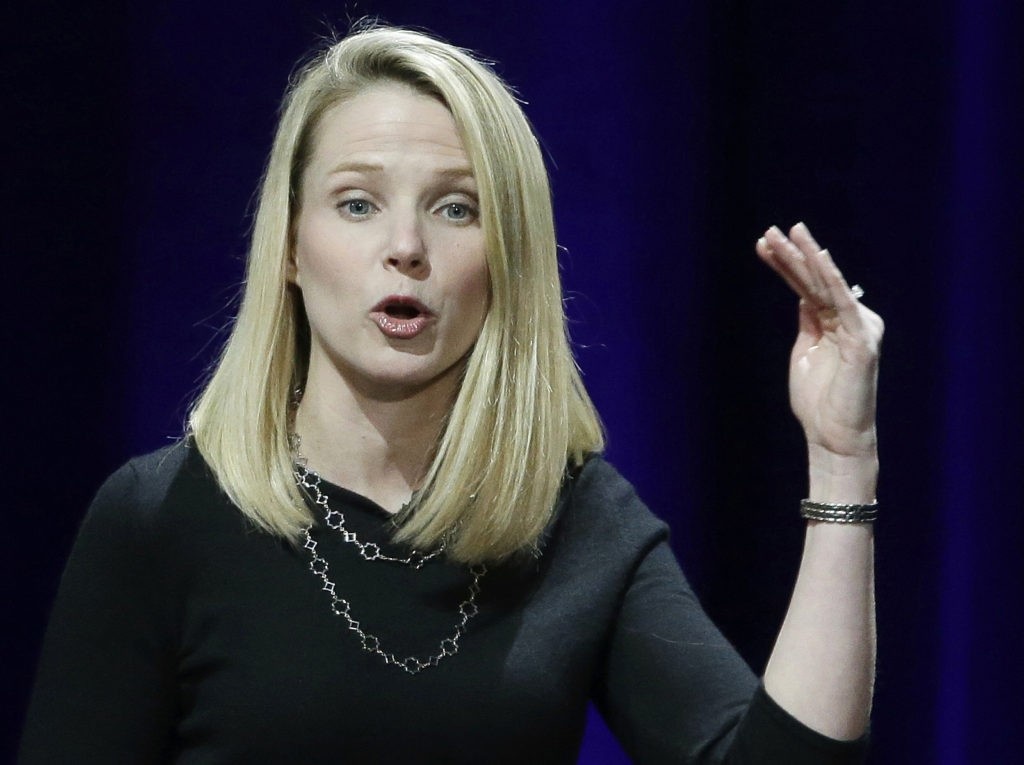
[817,284,865,312]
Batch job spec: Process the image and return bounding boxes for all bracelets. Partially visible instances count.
[795,495,883,524]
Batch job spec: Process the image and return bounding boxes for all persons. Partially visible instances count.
[14,24,886,765]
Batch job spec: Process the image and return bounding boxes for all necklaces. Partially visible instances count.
[277,380,507,674]
